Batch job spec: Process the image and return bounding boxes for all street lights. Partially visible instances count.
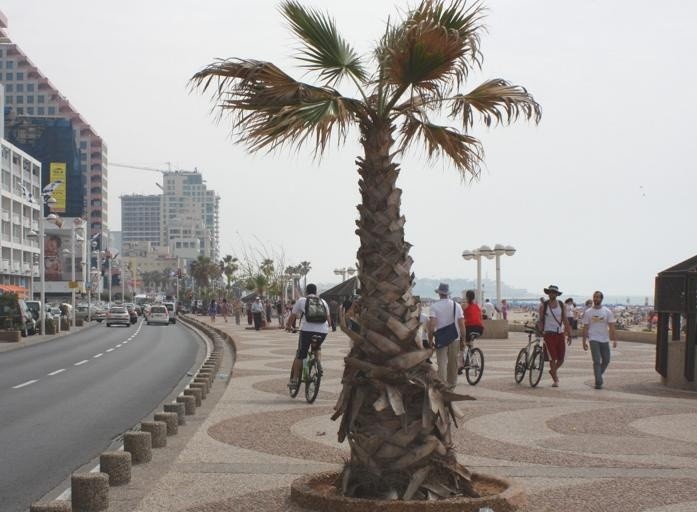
[28,191,60,337]
[169,272,228,297]
[62,222,131,328]
[333,266,357,282]
[462,245,491,308]
[279,273,292,303]
[288,271,301,302]
[485,244,516,320]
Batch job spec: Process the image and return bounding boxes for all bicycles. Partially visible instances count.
[513,322,545,388]
[456,331,485,386]
[283,326,323,405]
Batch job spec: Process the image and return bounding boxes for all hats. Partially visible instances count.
[435,282,450,295]
[544,285,561,296]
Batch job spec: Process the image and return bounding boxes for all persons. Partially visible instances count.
[209,295,295,331]
[287,282,362,389]
[414,281,616,390]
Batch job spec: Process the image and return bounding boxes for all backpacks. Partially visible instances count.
[305,296,327,323]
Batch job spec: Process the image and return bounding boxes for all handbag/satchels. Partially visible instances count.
[433,323,458,348]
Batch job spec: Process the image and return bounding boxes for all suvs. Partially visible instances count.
[1,299,36,337]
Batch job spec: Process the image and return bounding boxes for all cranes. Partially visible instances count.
[109,159,171,176]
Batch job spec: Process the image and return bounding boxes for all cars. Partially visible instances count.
[24,296,179,329]
